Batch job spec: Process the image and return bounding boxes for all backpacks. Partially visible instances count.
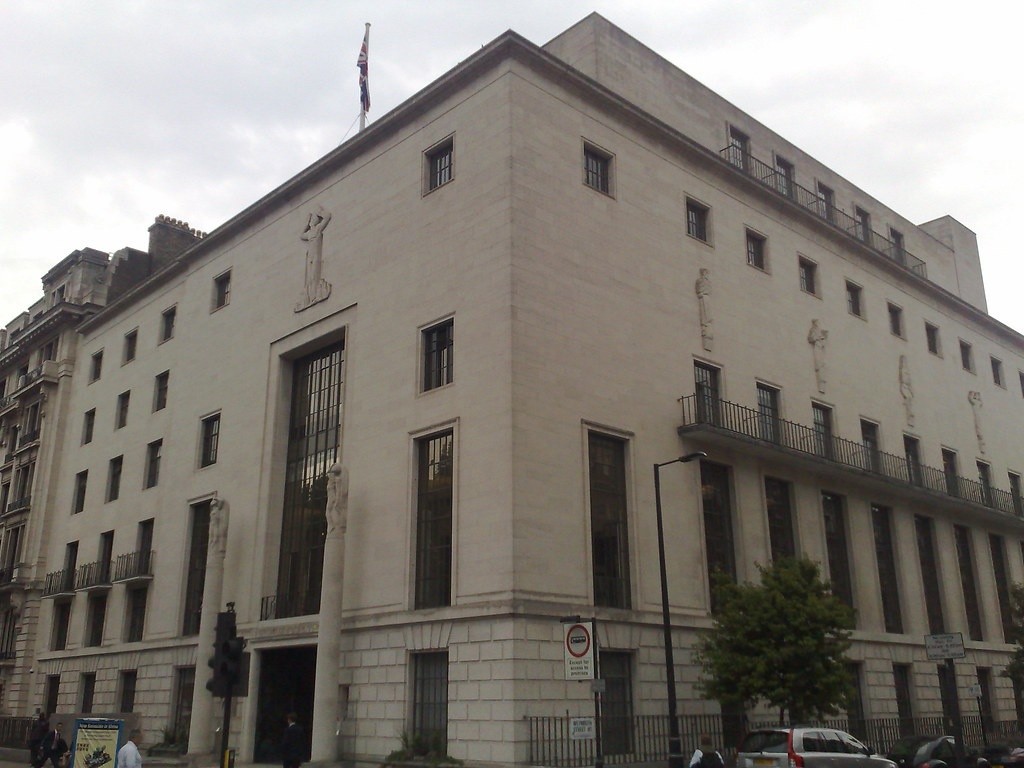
[697,748,723,768]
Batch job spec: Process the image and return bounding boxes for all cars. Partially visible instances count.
[734,726,1024,768]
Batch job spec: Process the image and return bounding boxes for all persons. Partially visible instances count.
[282,713,305,768]
[118,729,142,768]
[690,737,724,768]
[29,712,63,768]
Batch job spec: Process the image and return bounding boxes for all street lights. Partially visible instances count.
[653,451,709,768]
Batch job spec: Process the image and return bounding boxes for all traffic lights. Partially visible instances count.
[205,613,250,697]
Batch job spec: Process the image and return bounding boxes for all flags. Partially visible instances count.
[357,35,370,112]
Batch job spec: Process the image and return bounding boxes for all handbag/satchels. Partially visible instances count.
[37,748,48,763]
